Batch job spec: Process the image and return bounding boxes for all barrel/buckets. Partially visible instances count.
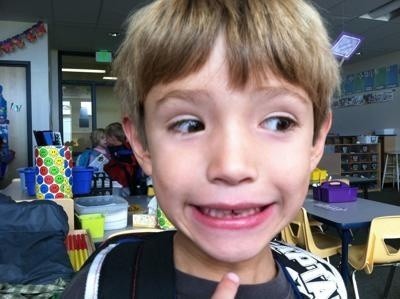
[72,165,98,194]
[23,166,36,196]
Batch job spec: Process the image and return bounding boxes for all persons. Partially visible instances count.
[87,127,111,173]
[100,121,138,189]
[55,0,356,299]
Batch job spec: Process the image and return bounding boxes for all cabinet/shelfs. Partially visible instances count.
[317,135,396,193]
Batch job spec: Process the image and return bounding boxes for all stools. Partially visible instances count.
[382,152,400,192]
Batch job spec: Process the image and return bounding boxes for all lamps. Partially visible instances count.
[78,107,89,127]
[331,31,365,68]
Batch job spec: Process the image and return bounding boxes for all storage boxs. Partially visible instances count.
[16,168,29,193]
[24,166,99,196]
[74,194,130,231]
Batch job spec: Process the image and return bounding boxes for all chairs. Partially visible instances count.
[281,207,400,299]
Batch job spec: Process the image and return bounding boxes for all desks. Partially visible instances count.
[3,178,148,247]
[302,189,400,287]
[327,175,377,199]
